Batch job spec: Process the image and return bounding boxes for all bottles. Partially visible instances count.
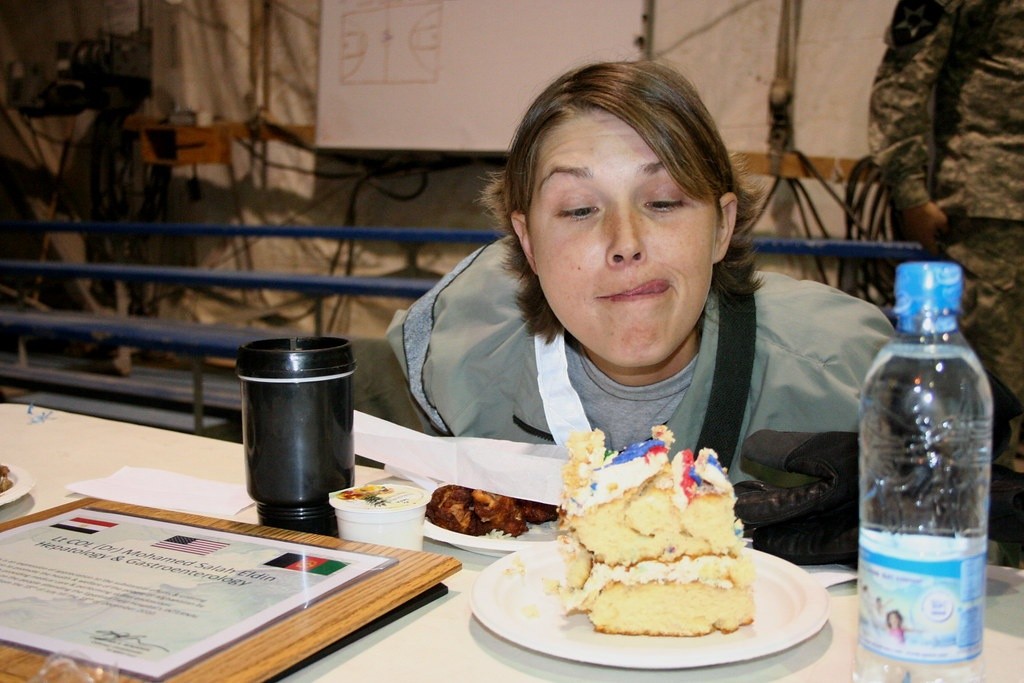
[851,261,993,683]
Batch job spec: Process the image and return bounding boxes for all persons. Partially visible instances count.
[385,61,894,489]
[876,610,919,648]
[869,0,1024,567]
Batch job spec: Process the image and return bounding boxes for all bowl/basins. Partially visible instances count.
[329,486,432,552]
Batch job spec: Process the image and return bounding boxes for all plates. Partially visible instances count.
[470,543,830,668]
[425,517,561,556]
[0,462,34,506]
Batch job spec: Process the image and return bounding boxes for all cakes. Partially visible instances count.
[558,422,757,637]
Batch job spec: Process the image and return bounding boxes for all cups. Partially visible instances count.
[237,336,358,538]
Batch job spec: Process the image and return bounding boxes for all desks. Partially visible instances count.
[0,404,1024,683]
